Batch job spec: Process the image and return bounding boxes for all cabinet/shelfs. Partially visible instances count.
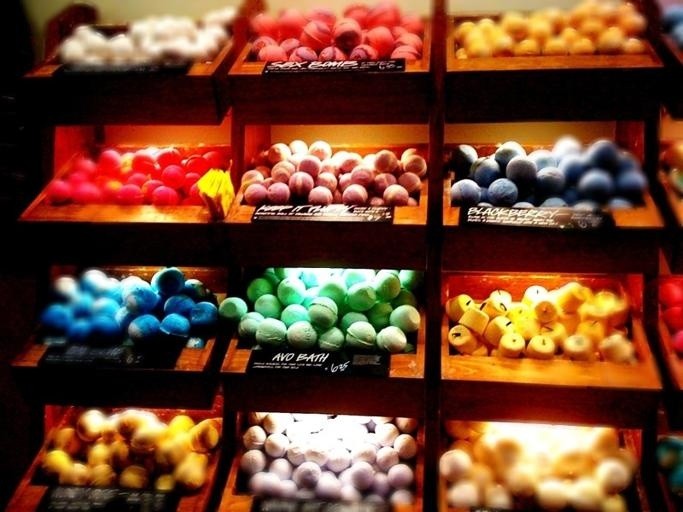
[0,2,683,511]
